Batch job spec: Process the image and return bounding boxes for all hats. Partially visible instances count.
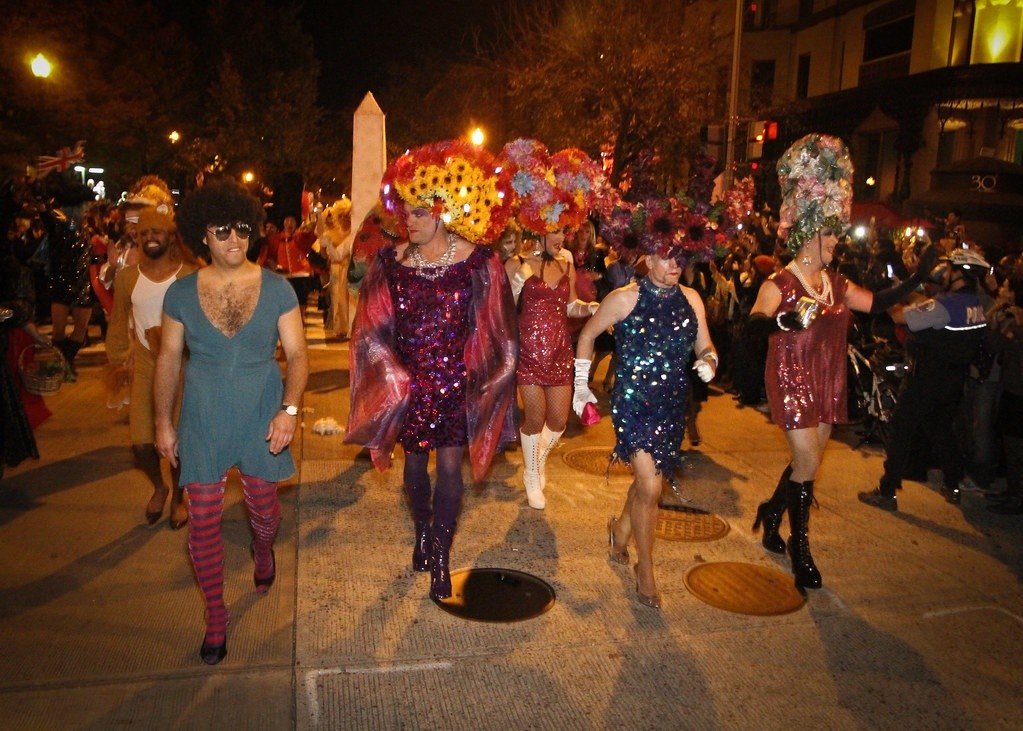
[938,249,992,276]
[118,183,171,206]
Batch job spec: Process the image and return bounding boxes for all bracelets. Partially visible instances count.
[705,353,718,368]
[777,312,790,331]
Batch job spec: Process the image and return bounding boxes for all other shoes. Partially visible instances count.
[717,364,1022,515]
[685,420,701,447]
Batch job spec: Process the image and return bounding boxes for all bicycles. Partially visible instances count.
[841,334,902,451]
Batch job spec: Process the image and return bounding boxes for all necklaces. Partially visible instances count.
[788,260,834,307]
[409,233,457,280]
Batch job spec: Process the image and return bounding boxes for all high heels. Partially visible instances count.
[633,564,662,609]
[606,514,630,566]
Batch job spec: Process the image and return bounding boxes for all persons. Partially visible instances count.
[4,227,25,259]
[573,233,718,608]
[155,178,309,665]
[693,230,793,410]
[355,199,607,598]
[743,226,936,588]
[858,248,990,510]
[247,215,309,317]
[99,202,196,532]
[46,199,121,366]
[830,235,1023,514]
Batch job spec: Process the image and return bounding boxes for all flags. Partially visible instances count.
[30,137,85,176]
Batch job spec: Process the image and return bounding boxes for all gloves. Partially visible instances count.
[572,358,597,418]
[567,298,601,319]
[694,353,719,383]
[870,243,940,317]
[745,311,802,333]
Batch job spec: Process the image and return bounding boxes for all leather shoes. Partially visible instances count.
[253,548,276,594]
[145,486,168,526]
[200,626,228,666]
[170,508,189,531]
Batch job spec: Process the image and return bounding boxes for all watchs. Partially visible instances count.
[281,404,298,416]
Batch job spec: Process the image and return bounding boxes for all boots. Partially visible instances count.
[519,427,546,510]
[785,479,823,590]
[403,482,431,571]
[752,464,794,555]
[428,489,463,598]
[537,421,566,490]
[50,335,85,380]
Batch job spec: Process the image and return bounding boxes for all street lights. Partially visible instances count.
[30,51,55,164]
[170,129,184,203]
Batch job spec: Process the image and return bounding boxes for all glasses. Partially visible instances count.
[821,227,840,239]
[204,223,254,241]
